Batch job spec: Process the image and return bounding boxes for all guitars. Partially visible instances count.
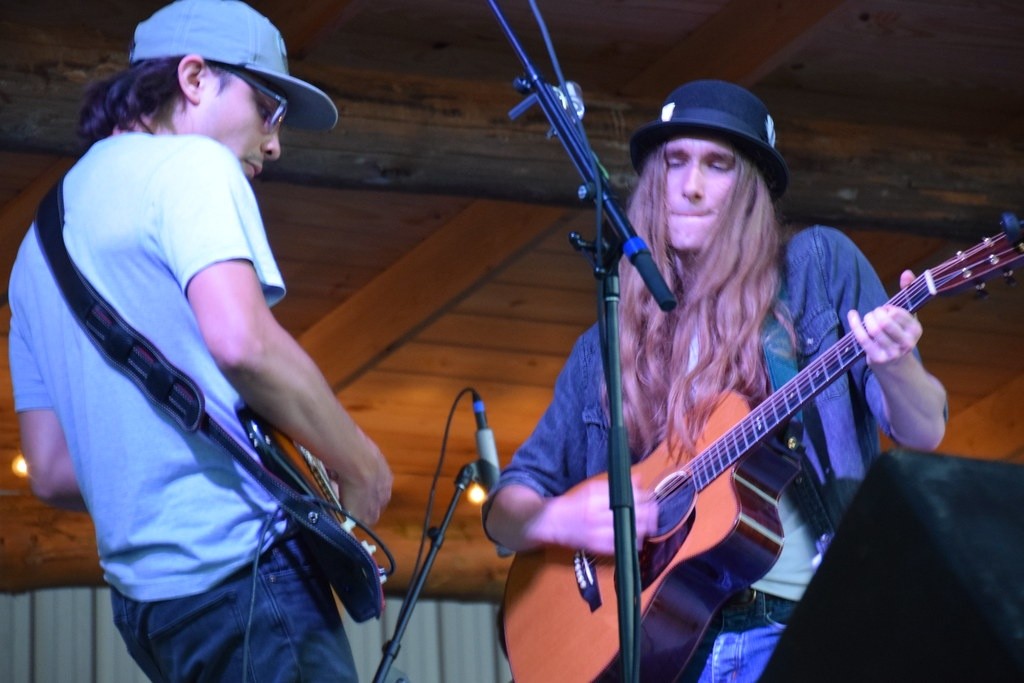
[240,410,387,622]
[498,214,1024,683]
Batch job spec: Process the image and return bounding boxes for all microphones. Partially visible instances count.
[469,395,511,558]
[560,80,584,125]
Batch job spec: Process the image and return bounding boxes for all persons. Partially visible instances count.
[5,0,396,683]
[481,77,955,683]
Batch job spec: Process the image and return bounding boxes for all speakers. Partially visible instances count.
[758,451,1024,682]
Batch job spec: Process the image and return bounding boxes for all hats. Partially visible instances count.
[131,1,339,132]
[631,79,789,200]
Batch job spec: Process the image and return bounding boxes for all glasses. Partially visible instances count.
[212,64,289,134]
